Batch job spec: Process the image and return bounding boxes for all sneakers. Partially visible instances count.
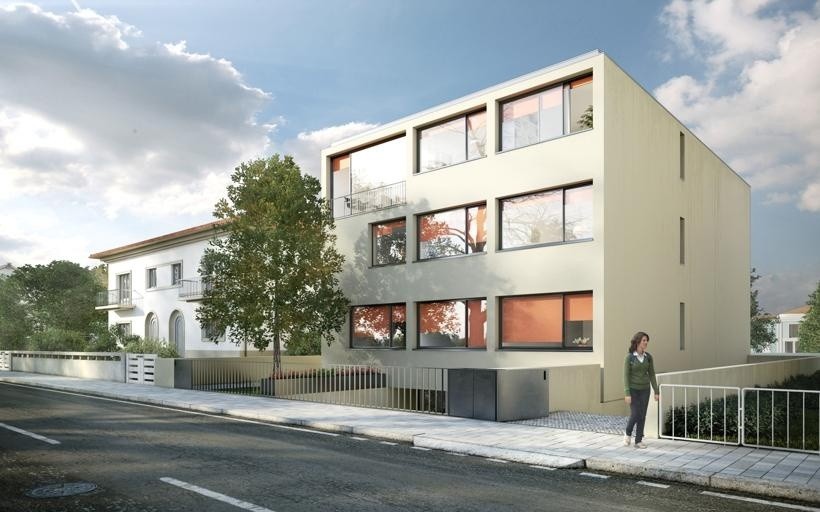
[624,432,648,449]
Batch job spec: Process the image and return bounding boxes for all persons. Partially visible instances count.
[622,331,659,448]
